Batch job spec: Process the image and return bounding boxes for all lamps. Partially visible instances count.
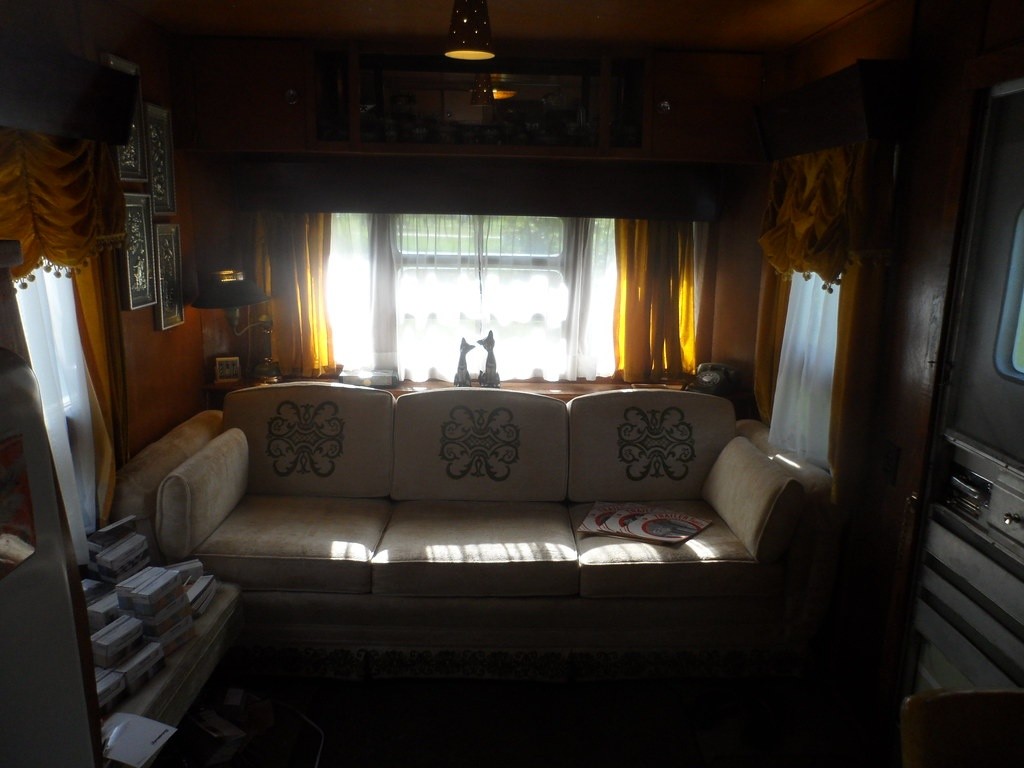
[442,0,497,61]
[192,269,277,339]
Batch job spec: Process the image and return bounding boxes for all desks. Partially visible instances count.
[99,585,247,768]
[202,381,270,412]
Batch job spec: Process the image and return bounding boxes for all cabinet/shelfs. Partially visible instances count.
[168,32,764,166]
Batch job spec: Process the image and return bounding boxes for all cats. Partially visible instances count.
[453,336,476,387]
[476,329,501,389]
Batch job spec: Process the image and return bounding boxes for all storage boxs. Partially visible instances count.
[80,514,219,713]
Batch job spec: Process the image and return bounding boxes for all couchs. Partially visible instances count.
[111,378,836,685]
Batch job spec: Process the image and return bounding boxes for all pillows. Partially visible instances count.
[699,435,804,561]
[157,427,248,563]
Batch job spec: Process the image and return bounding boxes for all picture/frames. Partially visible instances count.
[98,51,148,183]
[215,357,241,382]
[142,101,177,218]
[119,190,159,311]
[151,222,186,331]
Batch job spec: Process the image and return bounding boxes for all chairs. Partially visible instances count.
[899,687,1024,768]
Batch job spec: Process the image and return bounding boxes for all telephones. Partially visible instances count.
[686,362,733,393]
